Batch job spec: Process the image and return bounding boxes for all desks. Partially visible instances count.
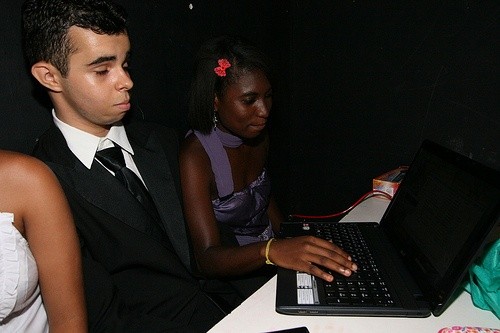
[205,193,500,333]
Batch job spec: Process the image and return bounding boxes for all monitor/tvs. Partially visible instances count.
[276,138,499,317]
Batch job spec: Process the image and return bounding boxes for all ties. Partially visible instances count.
[97,148,169,241]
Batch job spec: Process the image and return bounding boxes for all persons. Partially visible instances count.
[0,150,89,333]
[10,1,248,333]
[175,36,361,285]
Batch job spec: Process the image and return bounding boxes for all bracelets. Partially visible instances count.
[263,237,274,266]
